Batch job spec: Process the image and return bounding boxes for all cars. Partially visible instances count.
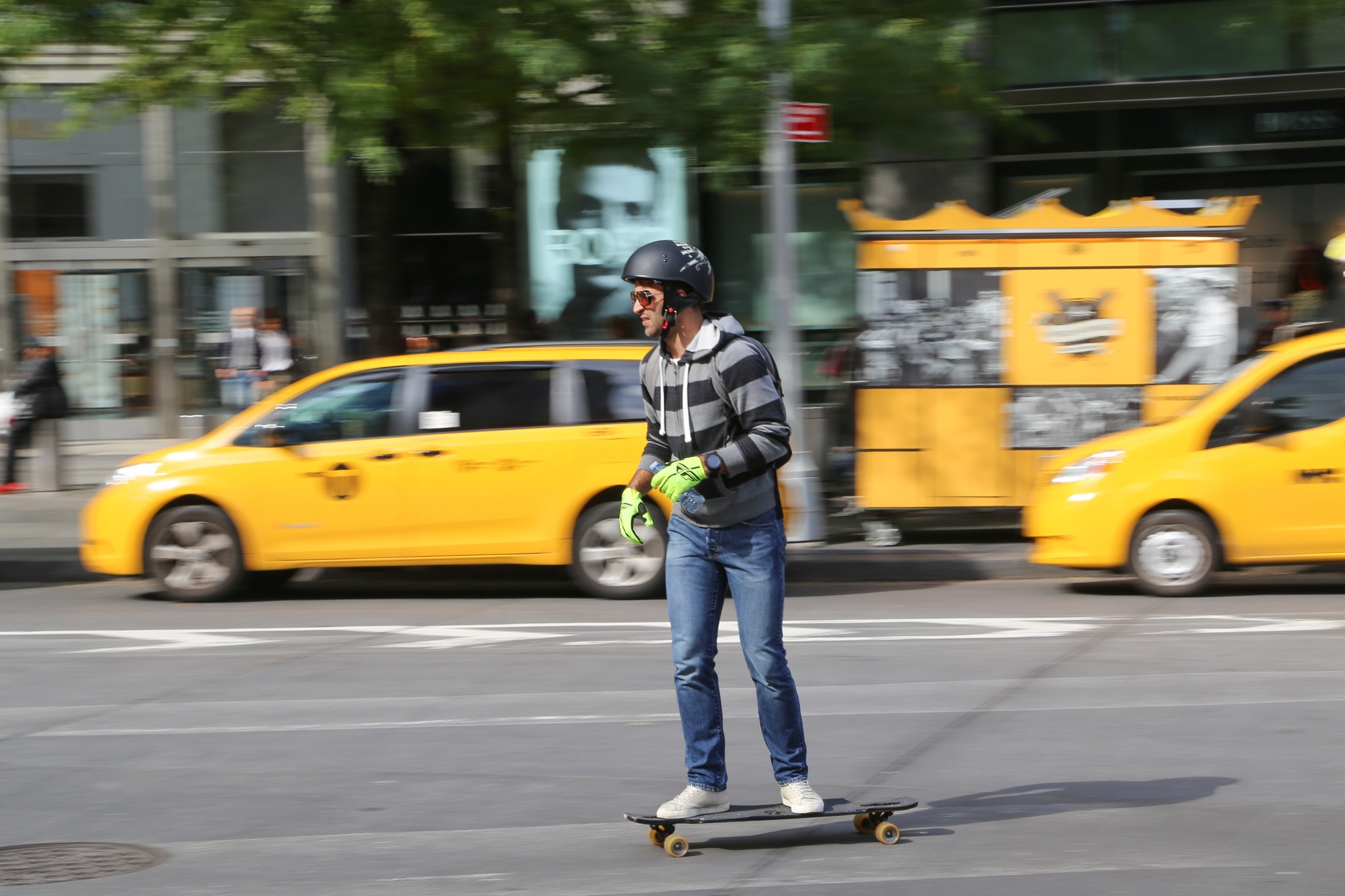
[1018,326,1345,598]
[77,340,791,602]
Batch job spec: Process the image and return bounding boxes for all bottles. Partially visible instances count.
[649,461,706,514]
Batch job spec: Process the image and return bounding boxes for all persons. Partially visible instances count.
[213,301,305,411]
[534,137,659,342]
[0,335,70,492]
[616,238,826,822]
[1154,273,1235,381]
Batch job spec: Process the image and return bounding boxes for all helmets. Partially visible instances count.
[622,239,714,304]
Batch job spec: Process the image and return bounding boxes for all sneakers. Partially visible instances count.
[656,783,730,821]
[780,778,825,814]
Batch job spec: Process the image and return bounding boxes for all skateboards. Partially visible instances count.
[623,797,918,858]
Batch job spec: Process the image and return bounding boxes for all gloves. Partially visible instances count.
[650,456,707,503]
[619,487,653,545]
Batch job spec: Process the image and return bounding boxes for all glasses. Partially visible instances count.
[629,289,664,307]
